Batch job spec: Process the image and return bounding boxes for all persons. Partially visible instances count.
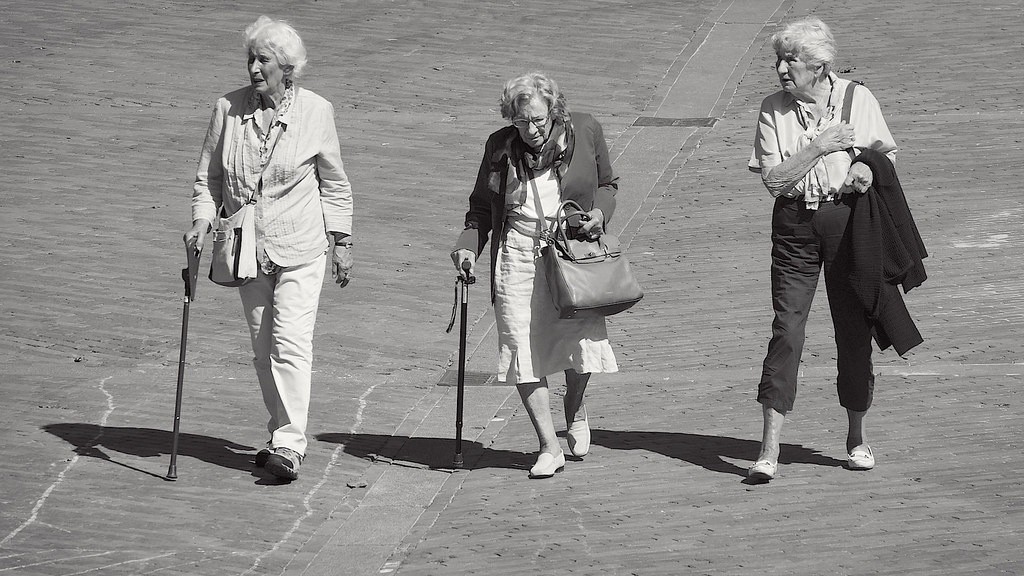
[182,17,353,481]
[746,18,896,480]
[450,73,618,476]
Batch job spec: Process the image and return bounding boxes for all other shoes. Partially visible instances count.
[847,444,875,470]
[563,391,591,457]
[748,460,778,479]
[530,448,565,479]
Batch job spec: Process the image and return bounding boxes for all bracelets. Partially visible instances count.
[335,242,352,249]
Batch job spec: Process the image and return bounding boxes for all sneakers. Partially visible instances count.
[265,447,301,479]
[255,439,276,468]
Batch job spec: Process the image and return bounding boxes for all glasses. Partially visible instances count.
[513,113,550,129]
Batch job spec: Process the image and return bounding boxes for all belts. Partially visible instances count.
[793,193,858,203]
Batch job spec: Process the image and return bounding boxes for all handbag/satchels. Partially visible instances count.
[208,203,257,288]
[541,200,644,319]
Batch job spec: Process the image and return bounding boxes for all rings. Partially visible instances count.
[850,136,853,140]
[589,232,593,237]
